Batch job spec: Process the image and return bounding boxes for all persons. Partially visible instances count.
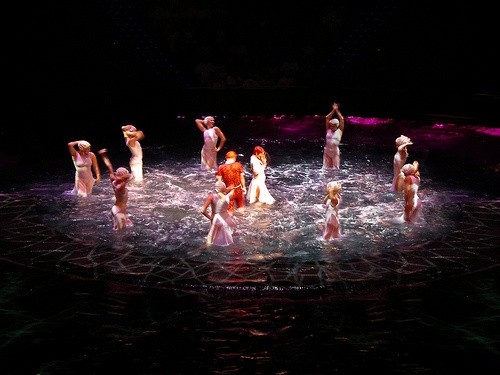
[68,141,100,196]
[395,161,420,220]
[100,148,131,230]
[196,116,226,170]
[201,181,234,245]
[246,146,270,202]
[215,151,247,215]
[324,181,341,239]
[322,103,344,169]
[122,124,146,184]
[390,135,413,190]
